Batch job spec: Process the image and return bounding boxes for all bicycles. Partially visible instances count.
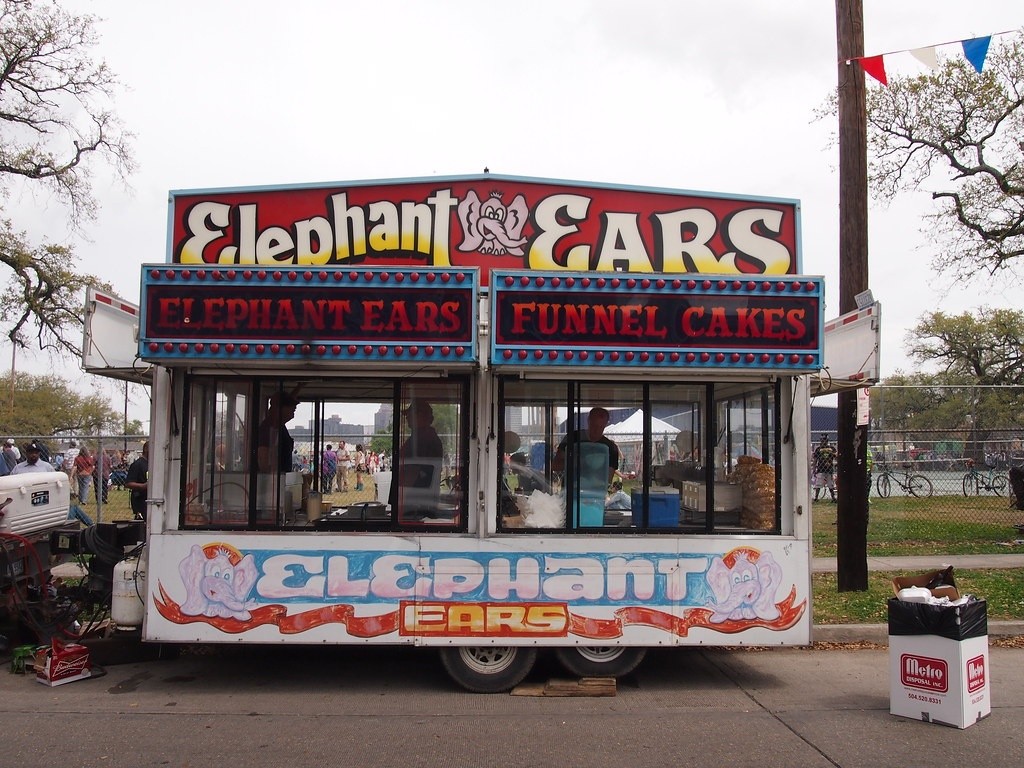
[811,462,837,502]
[876,463,933,498]
[963,459,1013,497]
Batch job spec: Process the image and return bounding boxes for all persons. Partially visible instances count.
[336,440,351,494]
[1,434,142,528]
[604,455,626,503]
[255,391,301,517]
[215,445,229,470]
[322,444,337,494]
[354,443,365,491]
[387,398,444,521]
[867,444,874,505]
[509,452,544,493]
[813,433,838,503]
[604,481,631,510]
[551,407,619,494]
[125,441,149,520]
[288,448,393,478]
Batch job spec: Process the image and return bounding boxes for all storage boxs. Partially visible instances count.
[679,481,743,523]
[0,472,70,536]
[888,570,959,600]
[11,635,92,687]
[630,486,680,527]
[563,442,609,528]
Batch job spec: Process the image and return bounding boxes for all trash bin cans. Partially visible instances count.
[887,593,992,730]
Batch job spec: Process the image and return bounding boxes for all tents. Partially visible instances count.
[603,407,682,473]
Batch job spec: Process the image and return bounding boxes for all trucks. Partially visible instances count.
[78,166,828,690]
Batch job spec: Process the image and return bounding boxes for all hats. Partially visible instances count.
[270,391,300,406]
[401,399,433,415]
[26,444,43,453]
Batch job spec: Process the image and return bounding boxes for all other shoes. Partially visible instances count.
[102,496,108,504]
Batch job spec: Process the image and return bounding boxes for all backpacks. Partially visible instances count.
[326,452,336,476]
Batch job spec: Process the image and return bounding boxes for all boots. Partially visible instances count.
[357,484,363,491]
[354,483,359,489]
